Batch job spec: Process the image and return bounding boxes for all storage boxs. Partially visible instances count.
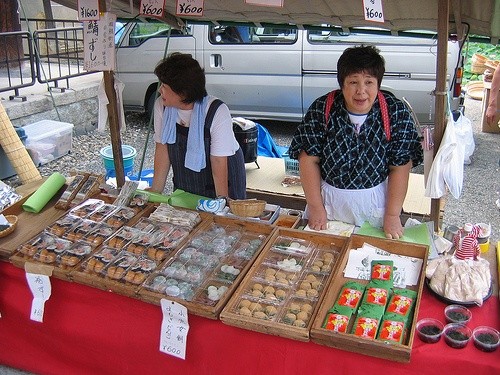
[0,170,430,364]
[21,119,74,168]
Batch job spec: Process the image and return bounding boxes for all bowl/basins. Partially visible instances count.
[416,318,445,344]
[129,169,154,187]
[443,305,472,324]
[472,326,500,351]
[443,323,472,349]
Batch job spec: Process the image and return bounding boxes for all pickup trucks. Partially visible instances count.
[116,20,468,127]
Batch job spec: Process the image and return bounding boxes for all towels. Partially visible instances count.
[160,92,212,173]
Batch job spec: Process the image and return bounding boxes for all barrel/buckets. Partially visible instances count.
[470,53,488,74]
[99,144,138,178]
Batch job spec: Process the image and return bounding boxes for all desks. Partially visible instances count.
[1,250,499,375]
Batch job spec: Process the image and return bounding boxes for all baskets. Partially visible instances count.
[229,198,267,217]
[281,149,299,176]
[470,53,499,81]
[0,214,18,238]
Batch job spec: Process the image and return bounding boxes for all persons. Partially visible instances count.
[143,52,246,203]
[485,63,500,211]
[288,45,424,239]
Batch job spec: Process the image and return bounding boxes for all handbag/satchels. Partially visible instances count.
[453,109,475,157]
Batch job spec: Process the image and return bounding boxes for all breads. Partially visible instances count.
[19,194,334,328]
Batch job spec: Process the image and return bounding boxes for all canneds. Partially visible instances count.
[473,223,491,244]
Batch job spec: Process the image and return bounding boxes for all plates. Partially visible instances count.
[425,276,494,305]
